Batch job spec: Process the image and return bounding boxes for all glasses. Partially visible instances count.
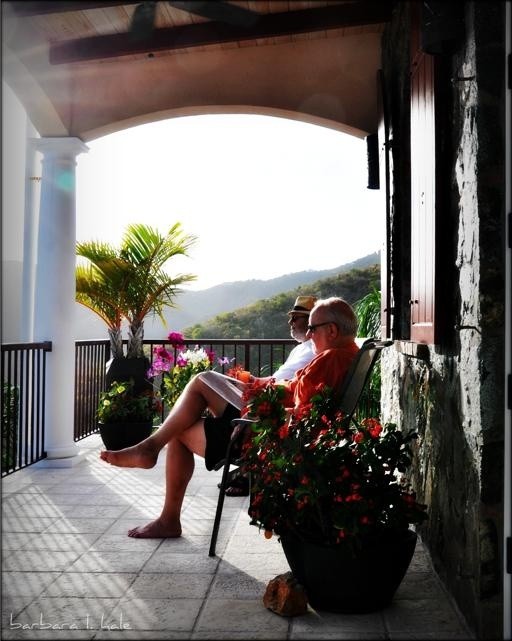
[292,315,305,321]
[308,322,328,332]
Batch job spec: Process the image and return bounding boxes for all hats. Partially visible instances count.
[287,297,318,317]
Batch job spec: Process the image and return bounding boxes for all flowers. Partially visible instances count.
[230,385,430,551]
[85,377,163,423]
[142,329,246,414]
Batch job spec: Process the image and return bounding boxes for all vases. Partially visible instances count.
[277,528,420,617]
[95,420,155,453]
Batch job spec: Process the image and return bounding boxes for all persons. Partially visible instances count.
[98,296,360,540]
[215,296,319,497]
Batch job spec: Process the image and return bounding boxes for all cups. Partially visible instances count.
[237,370,251,383]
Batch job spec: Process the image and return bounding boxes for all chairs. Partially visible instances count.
[351,337,377,353]
[205,339,399,559]
